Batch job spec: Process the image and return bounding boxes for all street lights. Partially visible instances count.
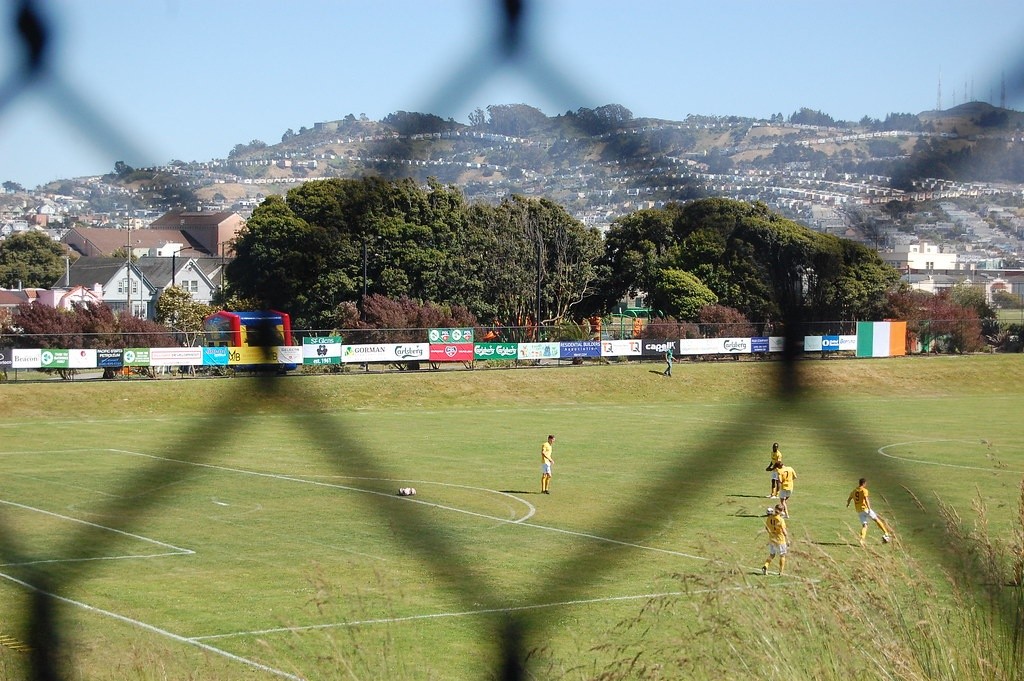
[363,235,383,321]
[171,246,194,324]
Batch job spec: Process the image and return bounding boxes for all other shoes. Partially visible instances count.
[762,566,768,575]
[783,516,790,519]
[541,490,549,494]
[882,535,890,543]
[766,494,779,500]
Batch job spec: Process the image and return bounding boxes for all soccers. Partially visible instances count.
[766,508,774,515]
[399,487,417,496]
[881,535,891,543]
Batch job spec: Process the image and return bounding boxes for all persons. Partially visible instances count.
[847,478,890,548]
[775,461,797,518]
[664,346,677,377]
[765,443,782,499]
[541,435,555,494]
[762,504,790,576]
